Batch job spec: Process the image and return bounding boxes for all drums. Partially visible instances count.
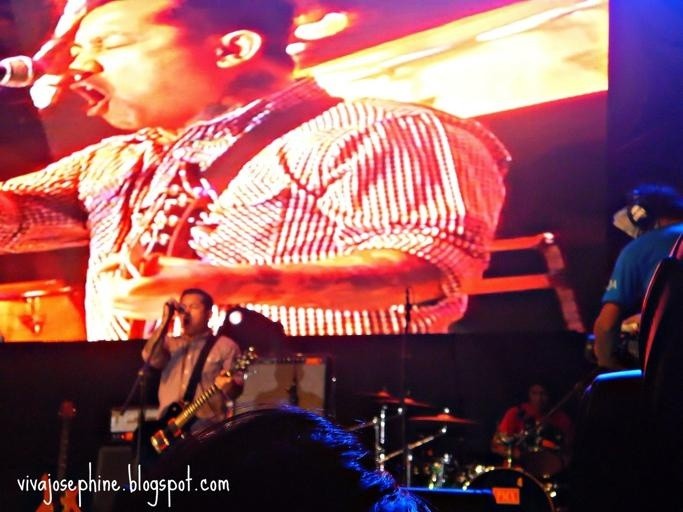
[519,427,566,480]
[462,466,557,512]
[0,281,88,347]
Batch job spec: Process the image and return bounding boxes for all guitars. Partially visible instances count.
[131,346,260,464]
[100,160,226,340]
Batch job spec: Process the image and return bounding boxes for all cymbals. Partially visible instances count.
[353,389,395,399]
[375,396,432,410]
[409,413,478,425]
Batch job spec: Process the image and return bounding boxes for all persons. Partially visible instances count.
[92,400,431,510]
[487,371,576,484]
[590,180,681,373]
[0,0,517,348]
[140,287,247,442]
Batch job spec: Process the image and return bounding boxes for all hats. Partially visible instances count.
[627,183,683,232]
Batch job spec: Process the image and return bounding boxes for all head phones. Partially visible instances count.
[626,189,657,231]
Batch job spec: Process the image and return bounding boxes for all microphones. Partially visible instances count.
[166,302,185,313]
[0,55,43,87]
